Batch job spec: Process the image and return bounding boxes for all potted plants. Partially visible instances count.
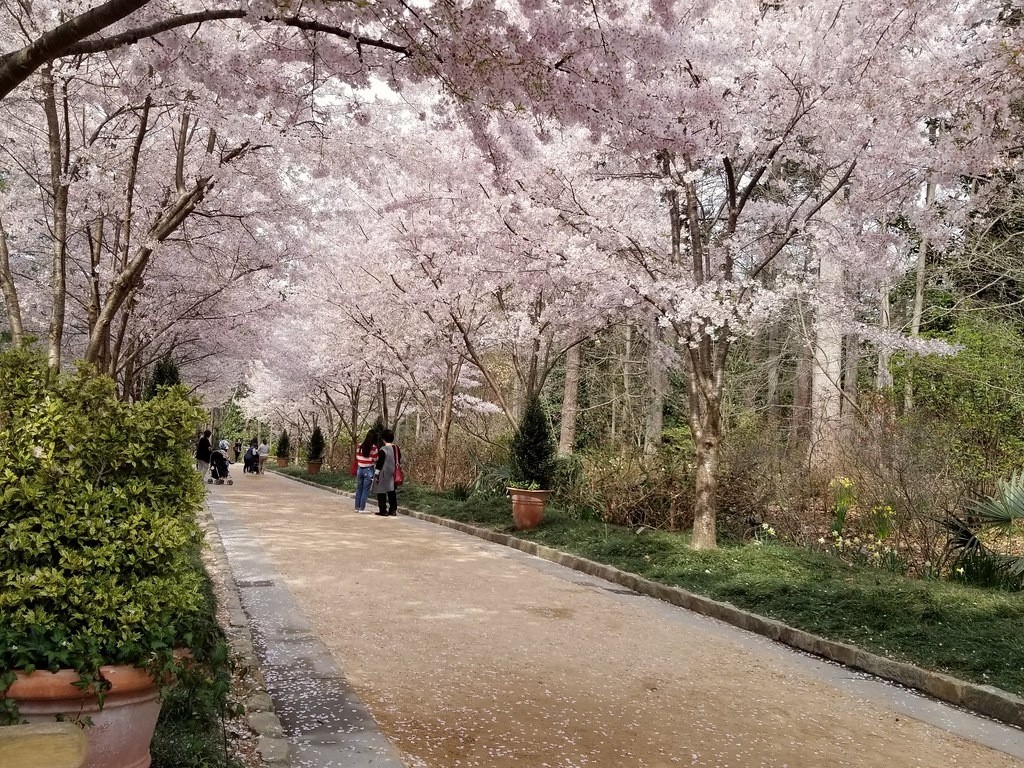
[307,427,325,474]
[507,395,556,531]
[274,429,290,468]
[0,350,210,768]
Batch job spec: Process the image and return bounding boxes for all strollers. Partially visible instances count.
[208,448,233,485]
[250,450,262,474]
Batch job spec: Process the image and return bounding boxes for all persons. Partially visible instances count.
[194,429,270,482]
[372,429,402,517]
[354,428,379,514]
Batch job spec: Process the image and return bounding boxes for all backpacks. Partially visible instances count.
[220,441,226,449]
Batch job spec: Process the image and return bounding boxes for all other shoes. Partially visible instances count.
[355,510,359,513]
[375,513,388,516]
[360,510,371,514]
[388,512,396,515]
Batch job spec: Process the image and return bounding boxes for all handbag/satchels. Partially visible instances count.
[349,459,358,475]
[395,445,404,485]
